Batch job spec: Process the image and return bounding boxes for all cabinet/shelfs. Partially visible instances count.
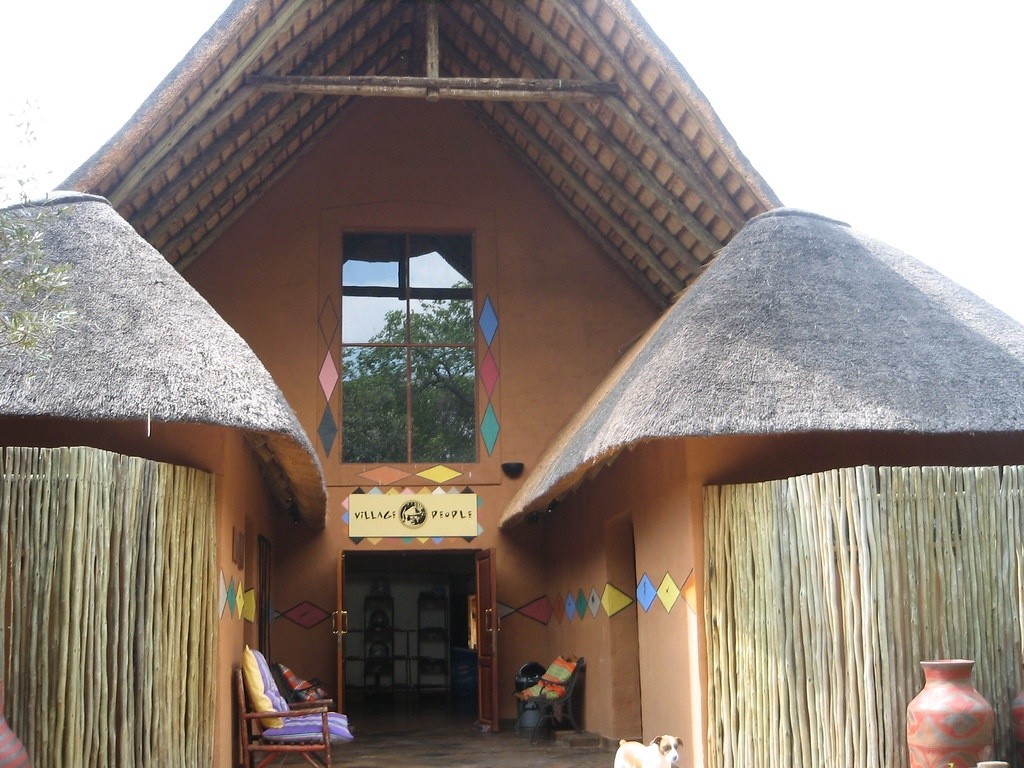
[346,596,448,693]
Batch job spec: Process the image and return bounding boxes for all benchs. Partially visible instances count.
[234,667,333,768]
[511,657,585,744]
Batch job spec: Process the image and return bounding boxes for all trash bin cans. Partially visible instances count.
[515,661,548,729]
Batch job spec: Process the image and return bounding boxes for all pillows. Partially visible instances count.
[242,645,289,728]
[273,660,328,702]
[260,711,354,746]
[538,655,578,686]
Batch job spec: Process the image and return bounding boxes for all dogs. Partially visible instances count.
[614,735,684,768]
